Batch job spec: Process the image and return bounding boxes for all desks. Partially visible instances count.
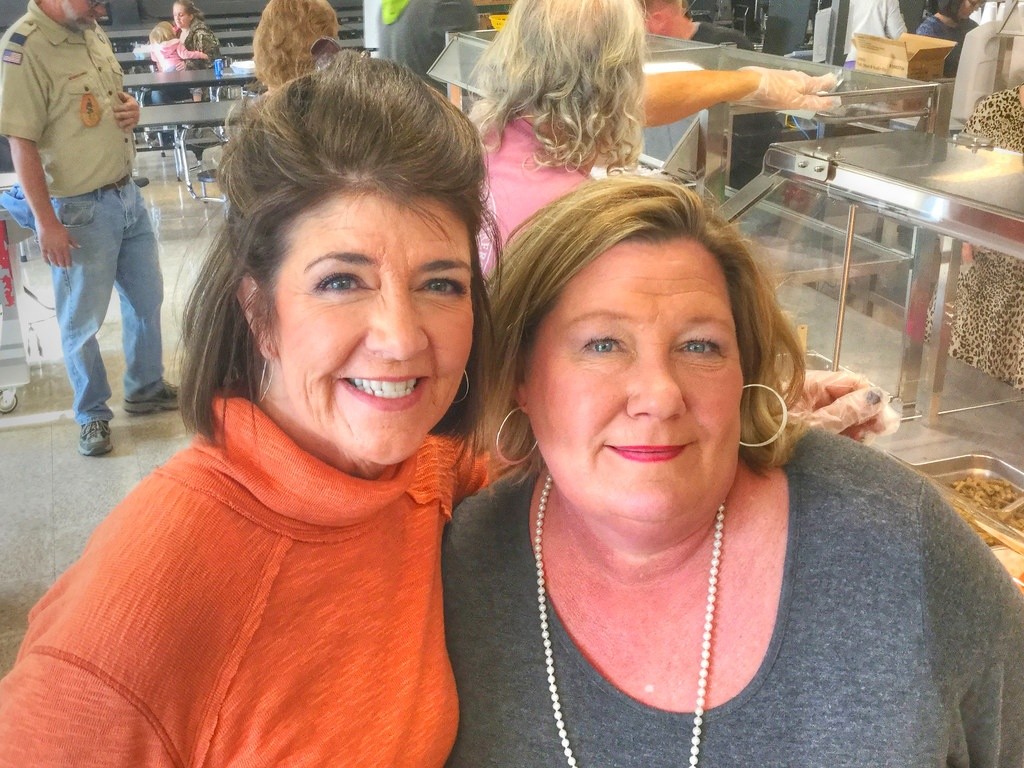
[103,6,363,201]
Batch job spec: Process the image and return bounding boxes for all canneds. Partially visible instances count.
[214,58,224,76]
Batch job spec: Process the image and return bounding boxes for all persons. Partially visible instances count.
[0,0,182,456]
[383,0,478,98]
[914,0,992,77]
[0,49,904,768]
[459,0,843,277]
[640,0,780,191]
[924,77,1024,388]
[143,0,219,106]
[150,20,211,103]
[442,176,1024,768]
[843,0,908,70]
[251,0,339,92]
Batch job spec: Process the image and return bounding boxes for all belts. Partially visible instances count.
[94,172,133,192]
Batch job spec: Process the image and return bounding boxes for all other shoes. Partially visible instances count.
[149,136,172,149]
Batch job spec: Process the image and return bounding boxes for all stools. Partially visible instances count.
[129,175,150,189]
[174,137,223,208]
[195,169,229,206]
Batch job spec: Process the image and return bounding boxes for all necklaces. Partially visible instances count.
[535,472,726,768]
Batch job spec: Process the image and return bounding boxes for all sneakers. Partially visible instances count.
[121,376,183,413]
[78,420,113,455]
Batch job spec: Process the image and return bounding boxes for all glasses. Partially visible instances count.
[88,0,110,8]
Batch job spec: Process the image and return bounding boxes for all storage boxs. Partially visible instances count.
[852,32,958,113]
